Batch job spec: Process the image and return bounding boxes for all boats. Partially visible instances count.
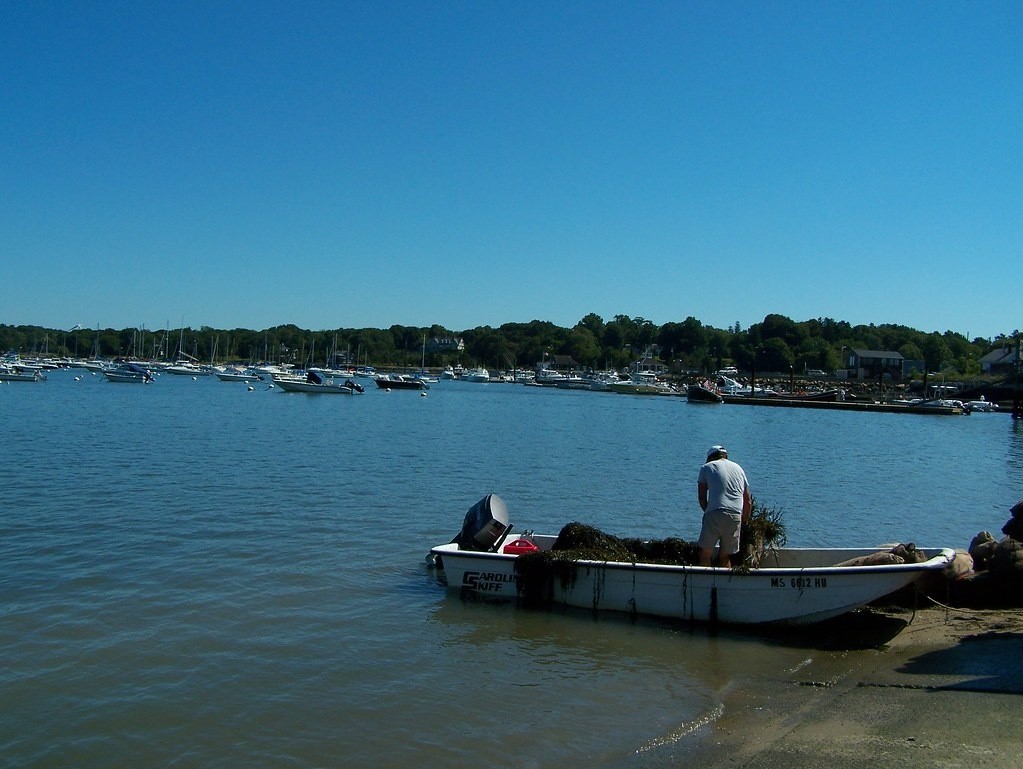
[606,361,671,394]
[893,384,999,412]
[373,334,440,391]
[686,384,723,403]
[717,375,845,402]
[440,349,622,392]
[423,494,956,632]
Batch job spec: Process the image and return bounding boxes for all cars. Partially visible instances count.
[718,367,738,376]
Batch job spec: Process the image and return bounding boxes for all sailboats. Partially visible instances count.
[0,315,382,393]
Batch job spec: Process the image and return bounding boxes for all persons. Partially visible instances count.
[697,445,752,567]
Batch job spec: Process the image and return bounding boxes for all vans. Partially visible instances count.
[808,369,828,377]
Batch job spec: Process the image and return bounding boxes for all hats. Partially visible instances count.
[706,445,727,462]
